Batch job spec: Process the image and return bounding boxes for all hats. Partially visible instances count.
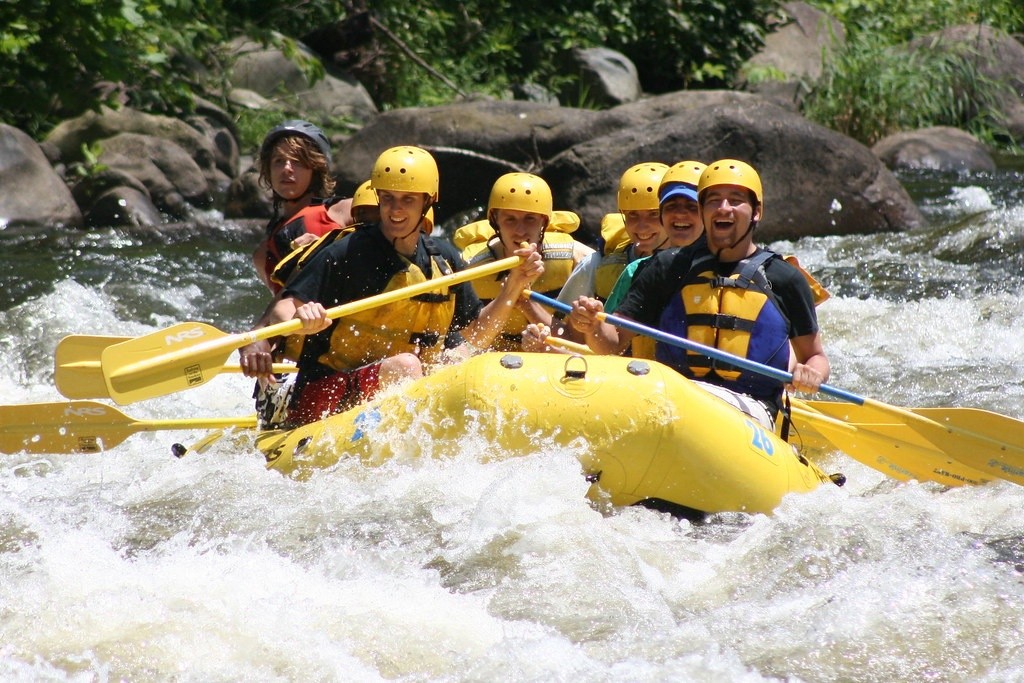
[659,184,698,205]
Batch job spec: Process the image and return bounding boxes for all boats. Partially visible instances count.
[253,350,847,528]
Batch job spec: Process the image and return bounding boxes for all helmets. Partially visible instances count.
[487,172,553,228]
[260,120,333,173]
[419,206,434,236]
[697,158,763,221]
[617,162,670,210]
[371,146,438,202]
[350,179,381,217]
[657,160,708,199]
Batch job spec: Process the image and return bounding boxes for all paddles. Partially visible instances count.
[518,285,1024,492]
[100,242,532,406]
[52,335,298,400]
[0,404,256,455]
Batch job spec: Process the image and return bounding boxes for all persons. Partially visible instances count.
[254,118,355,301]
[237,145,710,431]
[568,157,831,423]
